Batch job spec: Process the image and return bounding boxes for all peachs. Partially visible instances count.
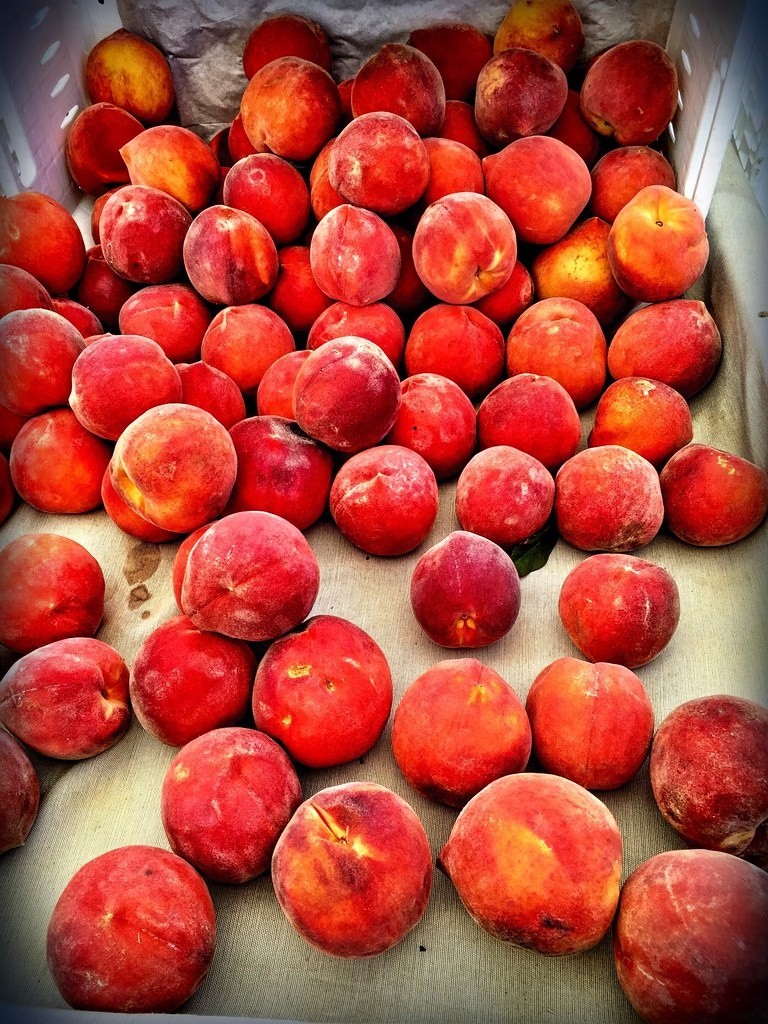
[0,0,768,1024]
